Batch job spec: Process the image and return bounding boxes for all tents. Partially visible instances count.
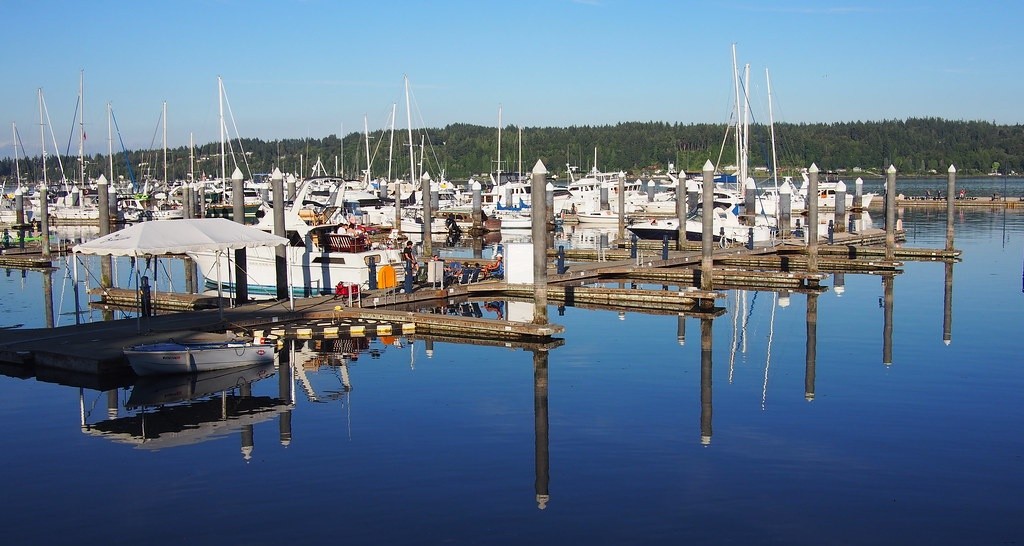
[74,218,294,334]
[79,383,296,446]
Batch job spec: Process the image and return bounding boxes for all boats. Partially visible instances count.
[124,362,276,413]
[122,342,275,381]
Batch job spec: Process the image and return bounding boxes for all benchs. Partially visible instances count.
[322,234,365,253]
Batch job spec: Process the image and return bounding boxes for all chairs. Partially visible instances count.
[436,302,483,318]
[443,261,504,286]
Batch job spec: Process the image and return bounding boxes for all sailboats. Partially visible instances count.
[2,57,642,309]
[629,48,779,244]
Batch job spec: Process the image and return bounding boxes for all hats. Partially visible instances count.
[495,253,502,257]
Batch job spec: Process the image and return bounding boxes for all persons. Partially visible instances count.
[484,254,502,274]
[338,223,370,244]
[927,188,931,196]
[475,263,479,268]
[434,255,438,261]
[938,189,942,198]
[458,262,468,275]
[450,213,454,220]
[31,218,39,232]
[960,189,965,198]
[404,241,419,272]
[482,301,503,320]
